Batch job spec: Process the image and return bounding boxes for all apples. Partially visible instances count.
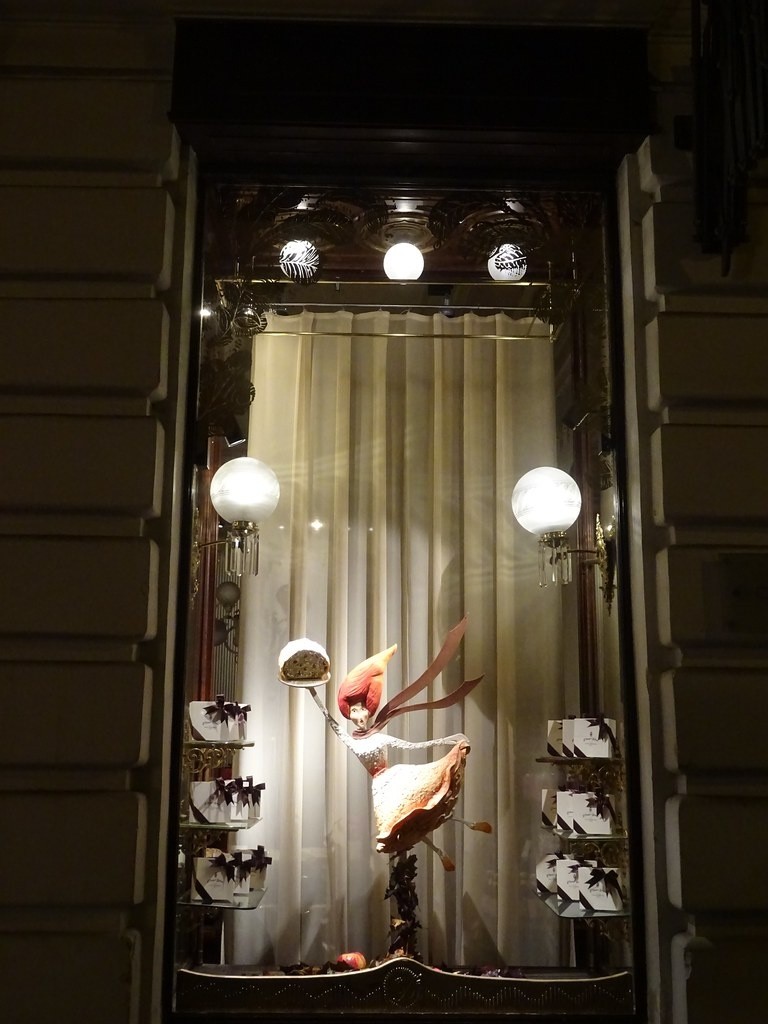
[336,952,367,969]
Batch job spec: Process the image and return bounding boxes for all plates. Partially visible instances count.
[277,669,332,688]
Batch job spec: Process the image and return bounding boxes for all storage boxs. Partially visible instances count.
[548,720,617,758]
[188,851,268,906]
[541,789,615,836]
[188,700,246,740]
[190,779,257,826]
[534,853,625,912]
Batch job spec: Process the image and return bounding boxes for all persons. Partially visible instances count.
[308,645,492,870]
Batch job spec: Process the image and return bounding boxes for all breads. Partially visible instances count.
[279,637,330,681]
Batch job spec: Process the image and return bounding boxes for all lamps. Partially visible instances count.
[189,455,282,592]
[381,222,428,281]
[280,230,320,278]
[513,467,614,615]
[481,225,530,280]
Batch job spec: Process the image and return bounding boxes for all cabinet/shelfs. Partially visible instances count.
[533,753,631,923]
[180,729,271,909]
